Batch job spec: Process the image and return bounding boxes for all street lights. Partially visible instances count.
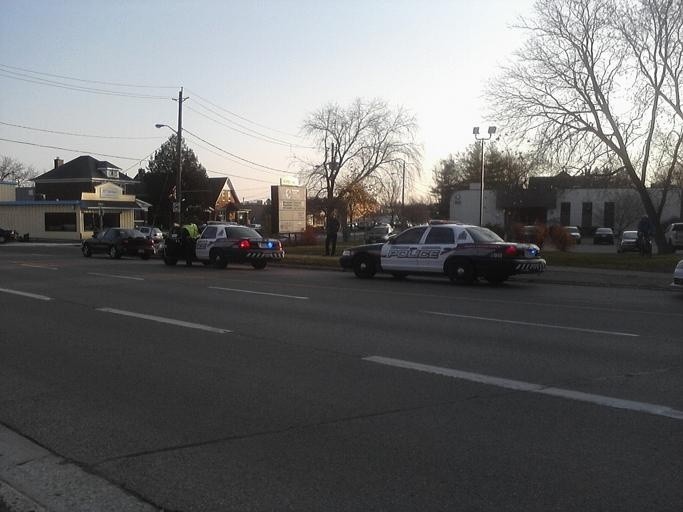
[155,122,184,228]
[471,126,496,227]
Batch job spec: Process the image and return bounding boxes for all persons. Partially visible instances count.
[636,215,650,243]
[322,212,341,256]
[181,218,200,265]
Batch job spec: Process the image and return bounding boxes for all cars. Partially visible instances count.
[671,259,682,294]
[334,220,547,285]
[0,227,14,244]
[521,217,681,257]
[74,220,284,271]
